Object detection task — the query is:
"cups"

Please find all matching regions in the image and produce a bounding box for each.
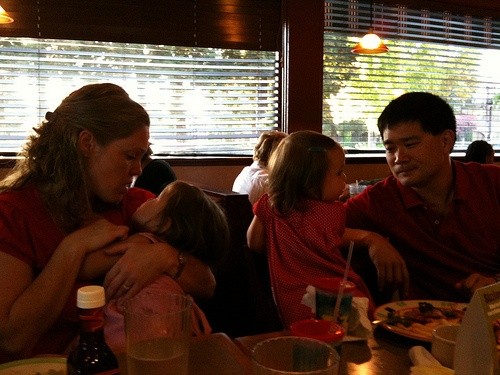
[251,337,340,375]
[431,323,461,369]
[122,294,190,375]
[310,278,356,335]
[349,184,366,198]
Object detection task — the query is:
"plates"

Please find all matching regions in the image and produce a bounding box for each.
[374,300,469,344]
[0,357,67,375]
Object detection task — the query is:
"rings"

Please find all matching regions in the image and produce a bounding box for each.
[121,283,132,290]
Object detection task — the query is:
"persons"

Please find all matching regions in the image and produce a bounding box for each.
[245,130,377,332]
[231,131,289,197]
[131,145,178,196]
[463,139,495,164]
[340,90,500,304]
[61,179,229,355]
[0,81,217,363]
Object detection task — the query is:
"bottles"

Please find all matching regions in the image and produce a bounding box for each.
[67,285,122,375]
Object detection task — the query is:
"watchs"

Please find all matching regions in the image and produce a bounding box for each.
[173,251,189,281]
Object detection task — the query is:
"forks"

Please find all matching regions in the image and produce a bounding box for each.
[372,311,432,326]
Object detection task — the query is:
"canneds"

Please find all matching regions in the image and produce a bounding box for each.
[291,318,346,372]
[314,277,355,325]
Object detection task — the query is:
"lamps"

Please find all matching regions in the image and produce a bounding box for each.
[0,4,14,24]
[349,0,388,54]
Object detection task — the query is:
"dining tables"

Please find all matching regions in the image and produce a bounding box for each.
[112,320,414,375]
